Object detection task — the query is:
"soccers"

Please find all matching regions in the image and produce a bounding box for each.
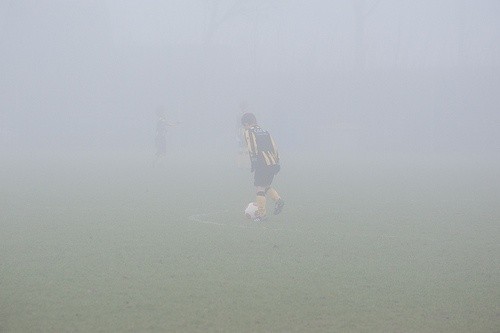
[245,201,264,220]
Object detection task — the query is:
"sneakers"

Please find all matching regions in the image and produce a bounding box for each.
[273,199,285,215]
[252,209,268,223]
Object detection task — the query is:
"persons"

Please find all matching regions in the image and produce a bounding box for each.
[241,113,285,223]
